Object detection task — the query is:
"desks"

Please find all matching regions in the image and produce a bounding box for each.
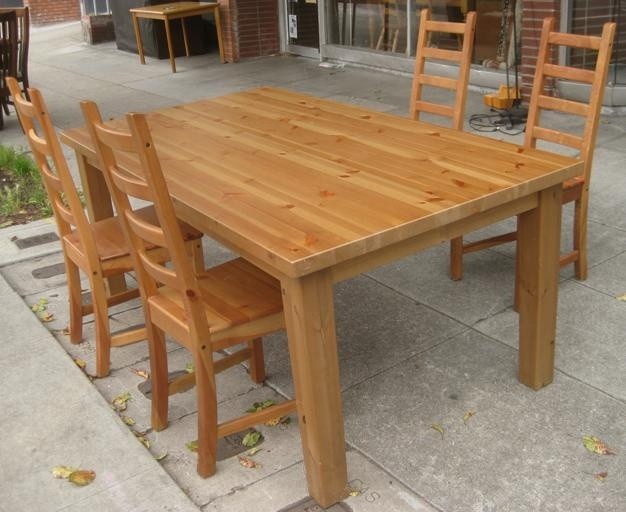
[128,2,226,74]
[57,86,586,509]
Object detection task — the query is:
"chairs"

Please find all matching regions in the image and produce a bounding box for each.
[375,0,433,52]
[0,6,32,130]
[80,99,297,478]
[407,8,476,132]
[449,15,615,312]
[7,77,206,379]
